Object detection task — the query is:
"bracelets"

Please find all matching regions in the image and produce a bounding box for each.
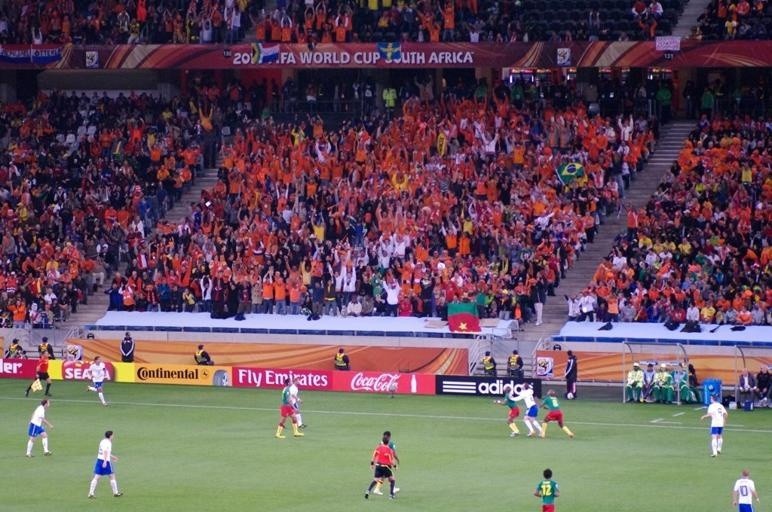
[756,498,759,501]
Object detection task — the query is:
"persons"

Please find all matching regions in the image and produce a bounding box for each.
[289,378,307,429]
[508,382,542,438]
[86,430,124,500]
[0,1,772,407]
[533,469,562,512]
[492,387,521,437]
[25,398,53,458]
[364,435,398,500]
[539,388,574,439]
[372,432,401,496]
[732,468,761,512]
[274,378,305,439]
[700,395,728,456]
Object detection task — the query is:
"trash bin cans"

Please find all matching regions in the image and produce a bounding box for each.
[704,379,723,405]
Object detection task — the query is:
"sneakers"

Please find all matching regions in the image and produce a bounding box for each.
[21,384,111,409]
[506,430,576,439]
[626,394,684,406]
[86,491,125,499]
[272,422,309,440]
[363,486,402,501]
[23,450,56,459]
[708,447,724,458]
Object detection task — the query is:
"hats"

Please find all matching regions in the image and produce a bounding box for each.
[631,361,685,369]
[12,337,48,344]
[125,332,130,336]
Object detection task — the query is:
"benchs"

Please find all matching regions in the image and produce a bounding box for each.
[541,379,737,390]
